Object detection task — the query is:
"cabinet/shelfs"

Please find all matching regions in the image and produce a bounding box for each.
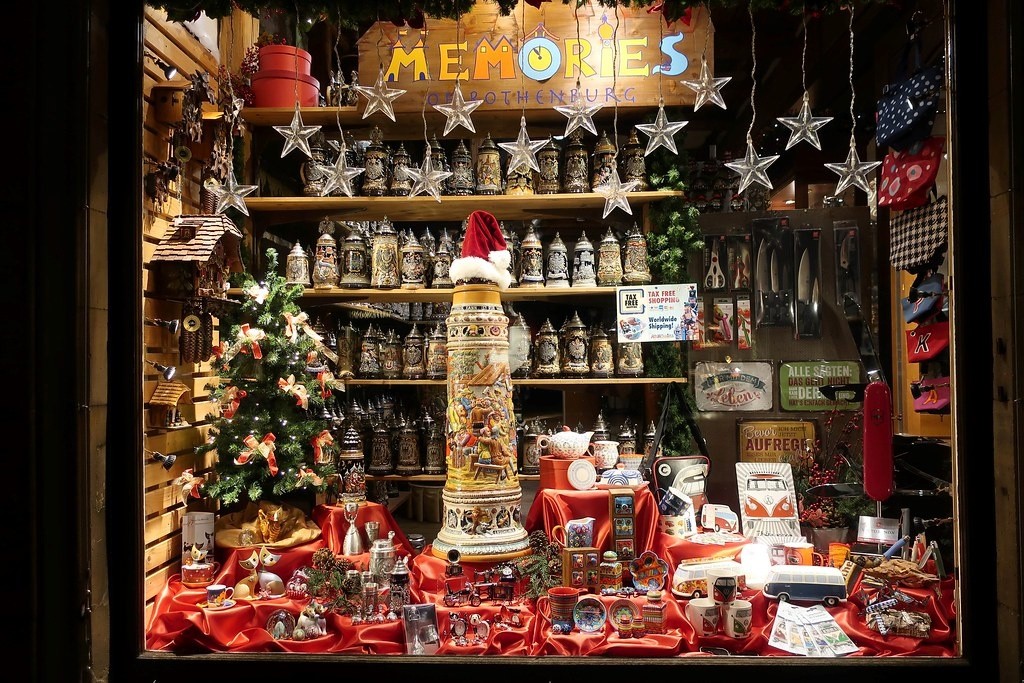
[144,1,969,670]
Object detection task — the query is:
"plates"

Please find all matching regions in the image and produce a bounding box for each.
[181,578,214,588]
[199,599,237,611]
[566,459,597,490]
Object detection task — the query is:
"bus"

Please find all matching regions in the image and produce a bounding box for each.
[672,562,747,599]
[763,566,848,608]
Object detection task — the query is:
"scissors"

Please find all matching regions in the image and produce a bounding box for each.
[705,238,725,288]
[728,241,750,289]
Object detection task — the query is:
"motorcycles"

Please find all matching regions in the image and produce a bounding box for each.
[443,582,481,607]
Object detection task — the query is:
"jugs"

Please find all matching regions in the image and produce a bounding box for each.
[537,426,595,460]
[587,441,620,468]
[551,517,595,547]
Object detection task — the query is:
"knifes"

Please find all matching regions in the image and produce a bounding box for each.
[756,238,819,336]
[838,239,849,298]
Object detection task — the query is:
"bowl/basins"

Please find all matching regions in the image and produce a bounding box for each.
[573,597,607,633]
[608,599,642,630]
[630,551,669,591]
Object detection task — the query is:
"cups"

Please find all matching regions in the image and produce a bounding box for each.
[784,542,824,566]
[658,515,685,540]
[206,585,234,608]
[619,454,644,470]
[706,568,737,606]
[537,588,580,634]
[364,522,380,544]
[723,600,753,639]
[181,562,220,583]
[684,598,719,637]
[654,487,693,516]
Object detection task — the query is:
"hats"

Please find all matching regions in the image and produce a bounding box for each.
[449,209,512,291]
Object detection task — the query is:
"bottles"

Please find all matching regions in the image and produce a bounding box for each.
[619,616,632,638]
[642,591,668,634]
[632,616,645,638]
[599,551,623,595]
